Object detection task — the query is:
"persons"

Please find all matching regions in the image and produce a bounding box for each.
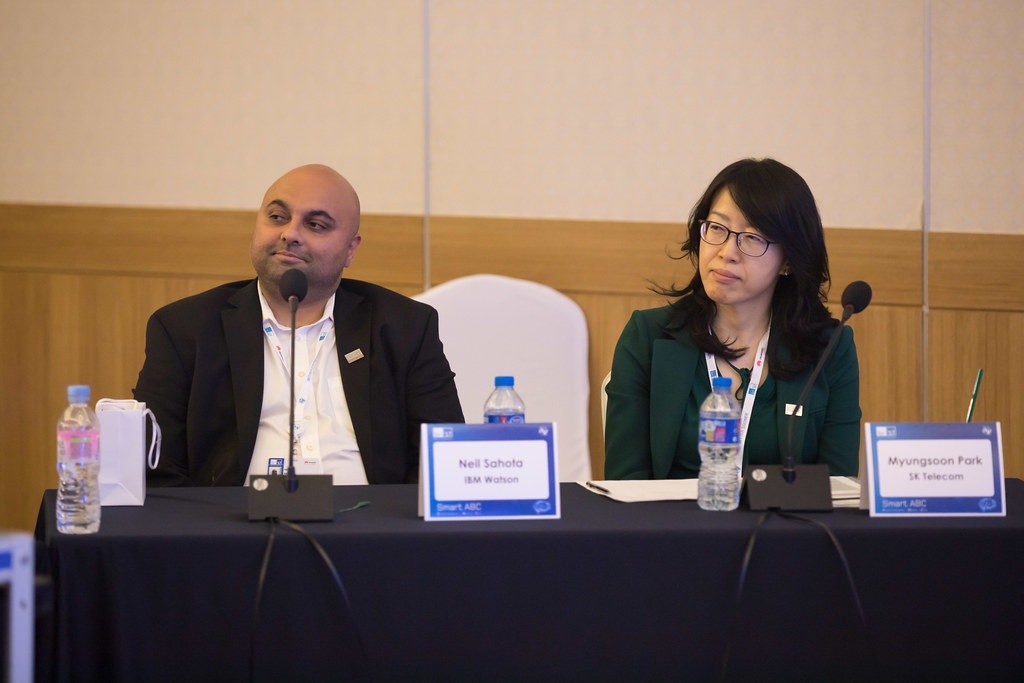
[603,158,863,482]
[130,163,465,487]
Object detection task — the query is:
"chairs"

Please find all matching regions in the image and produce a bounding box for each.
[407,271,591,483]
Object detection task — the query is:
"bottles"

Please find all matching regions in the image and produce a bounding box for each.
[696,378,746,511]
[54,385,103,534]
[483,377,526,426]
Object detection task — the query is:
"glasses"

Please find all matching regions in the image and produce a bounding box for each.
[698,219,783,257]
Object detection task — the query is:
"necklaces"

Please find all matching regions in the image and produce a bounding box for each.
[710,324,756,407]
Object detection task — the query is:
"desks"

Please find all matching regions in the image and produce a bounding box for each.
[33,475,1023,683]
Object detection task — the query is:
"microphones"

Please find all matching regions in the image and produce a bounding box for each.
[246,269,336,525]
[739,279,874,510]
